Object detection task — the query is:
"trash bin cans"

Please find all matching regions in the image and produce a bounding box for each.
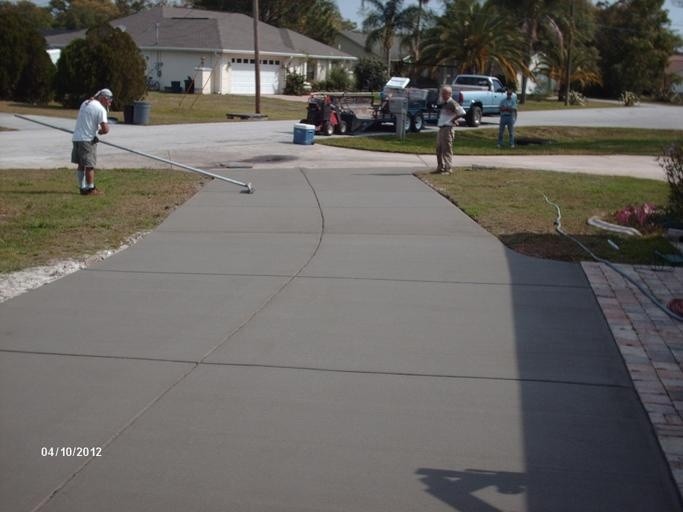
[124,100,150,124]
[172,81,180,93]
[184,80,194,93]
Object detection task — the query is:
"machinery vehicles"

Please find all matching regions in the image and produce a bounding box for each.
[298,85,428,136]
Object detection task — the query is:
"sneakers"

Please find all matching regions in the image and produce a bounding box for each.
[80,186,107,196]
[495,143,516,149]
[429,168,455,176]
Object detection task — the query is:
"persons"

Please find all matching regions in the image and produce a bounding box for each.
[429,87,466,175]
[495,86,519,149]
[71,87,115,196]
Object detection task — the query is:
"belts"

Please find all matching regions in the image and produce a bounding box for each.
[439,125,453,128]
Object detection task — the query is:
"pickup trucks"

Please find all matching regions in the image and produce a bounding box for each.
[424,75,517,127]
